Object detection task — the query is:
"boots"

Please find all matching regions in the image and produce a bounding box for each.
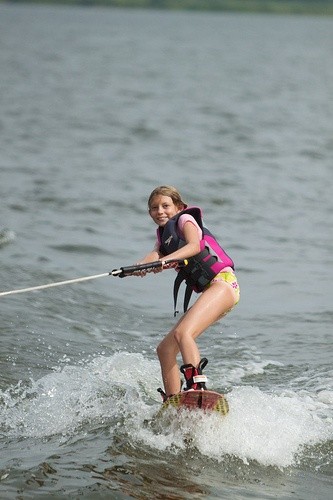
[179,358,208,392]
[157,380,183,402]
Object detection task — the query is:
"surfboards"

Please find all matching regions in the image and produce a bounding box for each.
[148,388,232,420]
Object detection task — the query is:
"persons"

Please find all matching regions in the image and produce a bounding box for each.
[125,184,242,403]
